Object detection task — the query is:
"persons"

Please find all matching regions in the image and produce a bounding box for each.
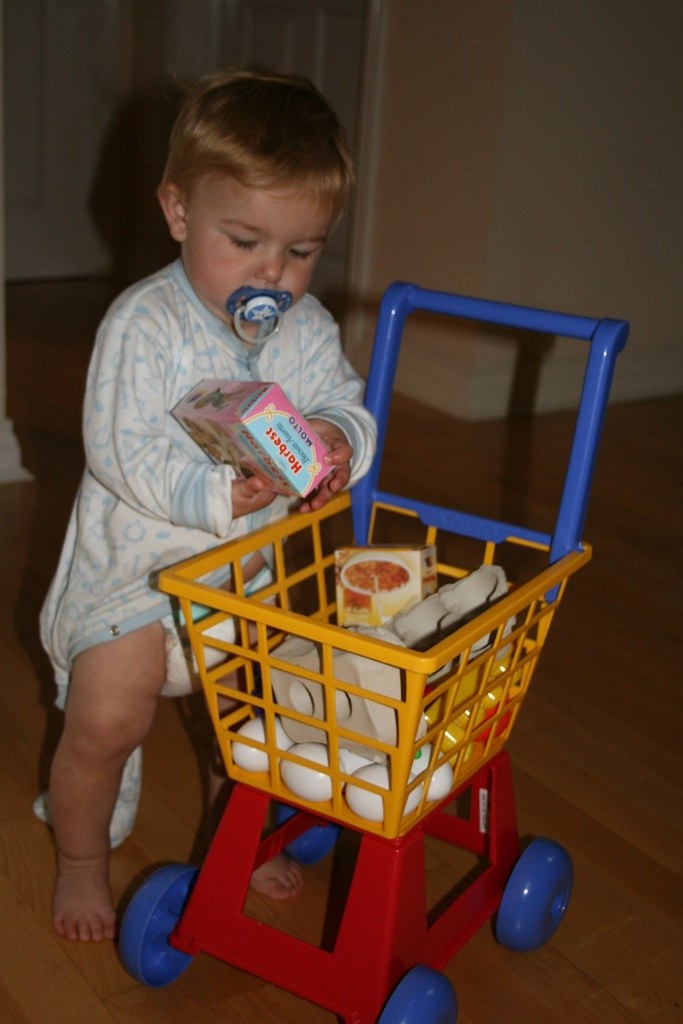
[35,64,376,943]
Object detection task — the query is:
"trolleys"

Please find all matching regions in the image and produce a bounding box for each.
[117,270,631,1023]
